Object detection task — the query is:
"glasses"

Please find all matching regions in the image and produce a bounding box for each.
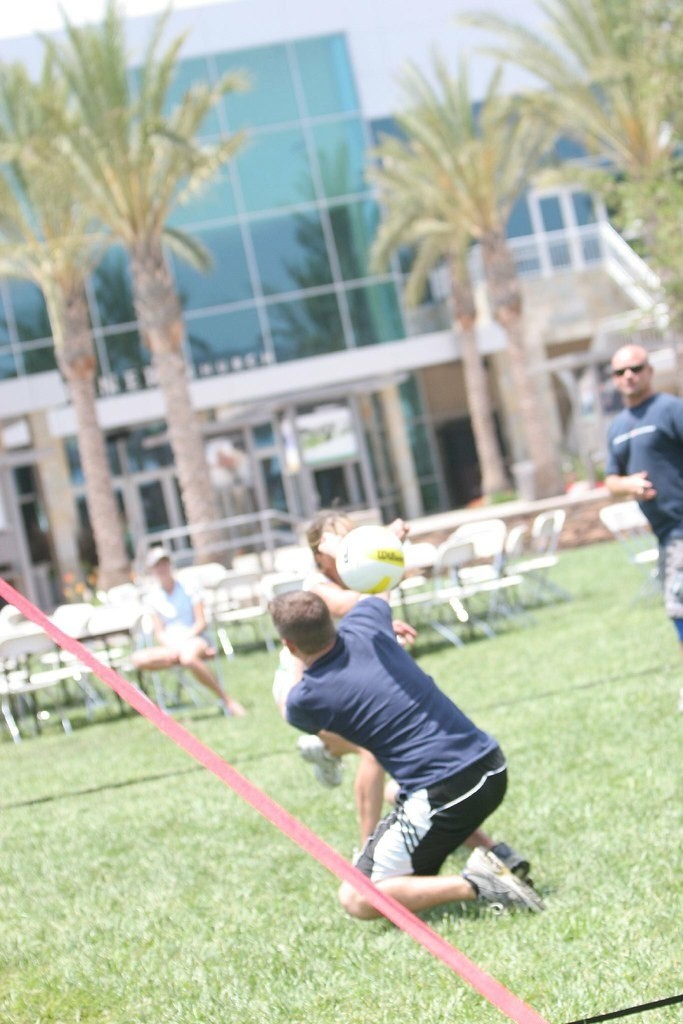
[613,364,646,376]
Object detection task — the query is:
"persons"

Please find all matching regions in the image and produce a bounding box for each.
[267,510,547,918]
[131,547,246,716]
[606,345,683,715]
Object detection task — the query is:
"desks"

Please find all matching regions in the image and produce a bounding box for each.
[25,628,150,737]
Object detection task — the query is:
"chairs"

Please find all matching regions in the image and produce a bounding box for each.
[0,508,567,742]
[599,499,663,591]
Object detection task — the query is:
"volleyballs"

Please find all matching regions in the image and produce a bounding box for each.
[334,523,408,596]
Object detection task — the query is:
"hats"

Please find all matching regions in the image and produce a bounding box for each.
[147,547,172,568]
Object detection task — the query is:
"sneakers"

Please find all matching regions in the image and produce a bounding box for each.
[489,841,534,888]
[460,844,547,916]
[296,735,344,790]
